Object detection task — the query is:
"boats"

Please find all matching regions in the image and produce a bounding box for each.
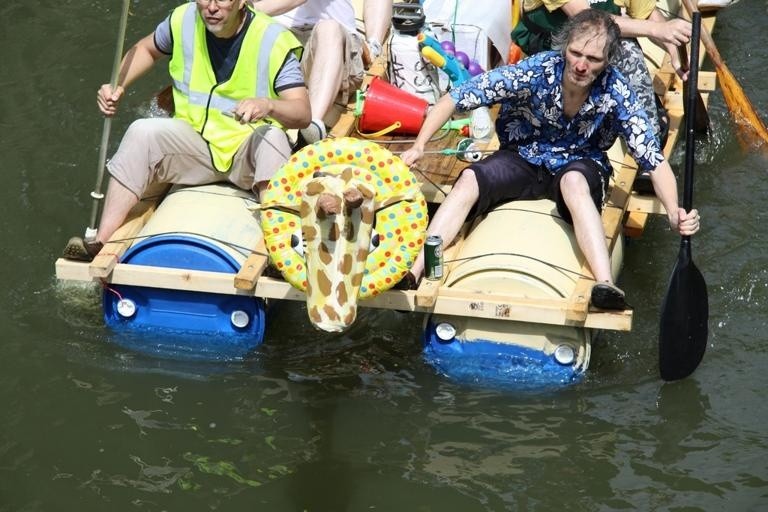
[55,3,721,397]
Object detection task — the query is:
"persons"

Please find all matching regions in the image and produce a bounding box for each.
[60,1,314,261]
[248,0,738,200]
[389,7,703,312]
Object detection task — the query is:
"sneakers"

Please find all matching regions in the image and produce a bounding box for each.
[590,282,626,310]
[296,119,326,147]
[62,237,103,262]
[391,272,417,290]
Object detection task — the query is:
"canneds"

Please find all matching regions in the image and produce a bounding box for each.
[455,138,482,163]
[424,235,444,281]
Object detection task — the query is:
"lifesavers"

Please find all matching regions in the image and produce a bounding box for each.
[260,137,429,334]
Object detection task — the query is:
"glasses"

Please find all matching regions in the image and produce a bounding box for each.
[196,0,233,6]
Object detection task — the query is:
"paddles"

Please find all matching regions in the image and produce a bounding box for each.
[676,36,713,136]
[682,0,768,156]
[660,13,708,380]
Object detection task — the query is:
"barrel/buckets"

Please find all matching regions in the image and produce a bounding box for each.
[423,273,591,393]
[103,239,267,361]
[353,74,428,140]
